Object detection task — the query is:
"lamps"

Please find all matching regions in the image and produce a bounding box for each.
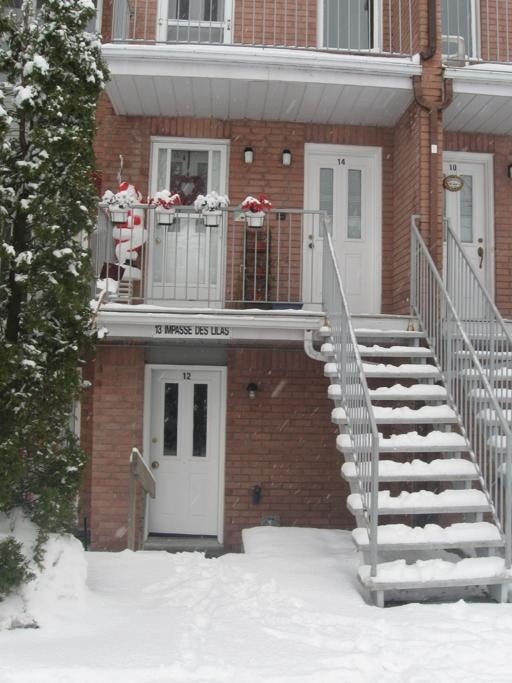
[246,383,257,399]
[282,150,291,166]
[244,147,253,163]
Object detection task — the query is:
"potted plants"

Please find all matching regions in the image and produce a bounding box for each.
[194,191,230,227]
[147,189,182,225]
[98,189,133,224]
[240,193,272,228]
